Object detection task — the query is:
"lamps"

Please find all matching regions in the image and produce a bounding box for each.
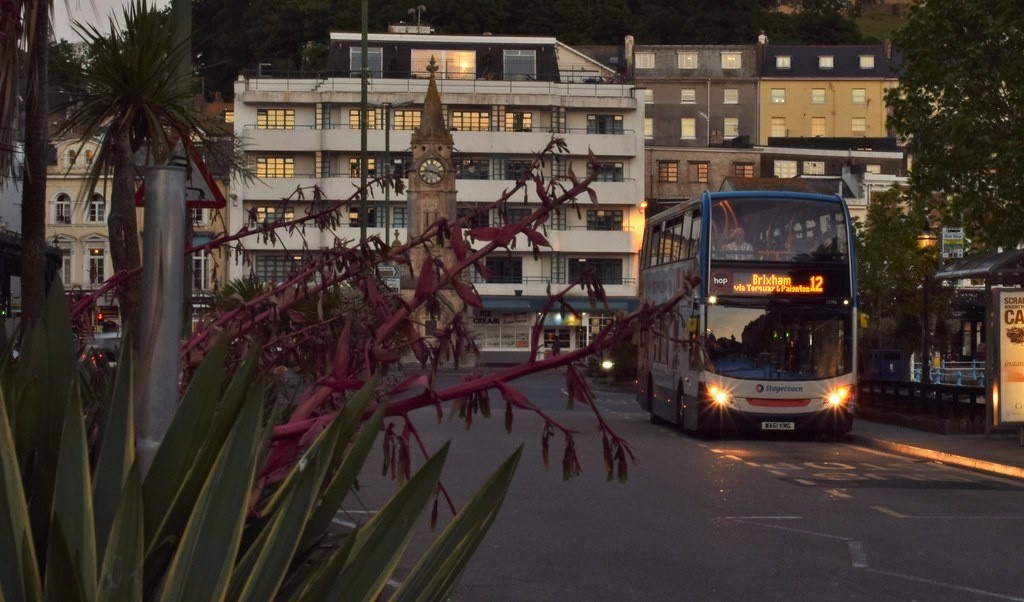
[230,192,239,207]
[640,200,647,214]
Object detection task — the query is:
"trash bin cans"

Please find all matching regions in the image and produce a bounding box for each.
[863,348,906,380]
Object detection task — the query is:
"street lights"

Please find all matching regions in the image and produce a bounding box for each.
[915,229,938,385]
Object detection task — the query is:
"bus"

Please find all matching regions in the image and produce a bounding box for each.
[633,189,860,438]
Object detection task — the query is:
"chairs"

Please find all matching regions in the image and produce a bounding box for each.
[789,239,815,260]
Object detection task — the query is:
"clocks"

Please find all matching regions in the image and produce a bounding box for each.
[418,158,445,184]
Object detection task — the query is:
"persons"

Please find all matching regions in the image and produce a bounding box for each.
[550,333,561,357]
[722,228,755,260]
